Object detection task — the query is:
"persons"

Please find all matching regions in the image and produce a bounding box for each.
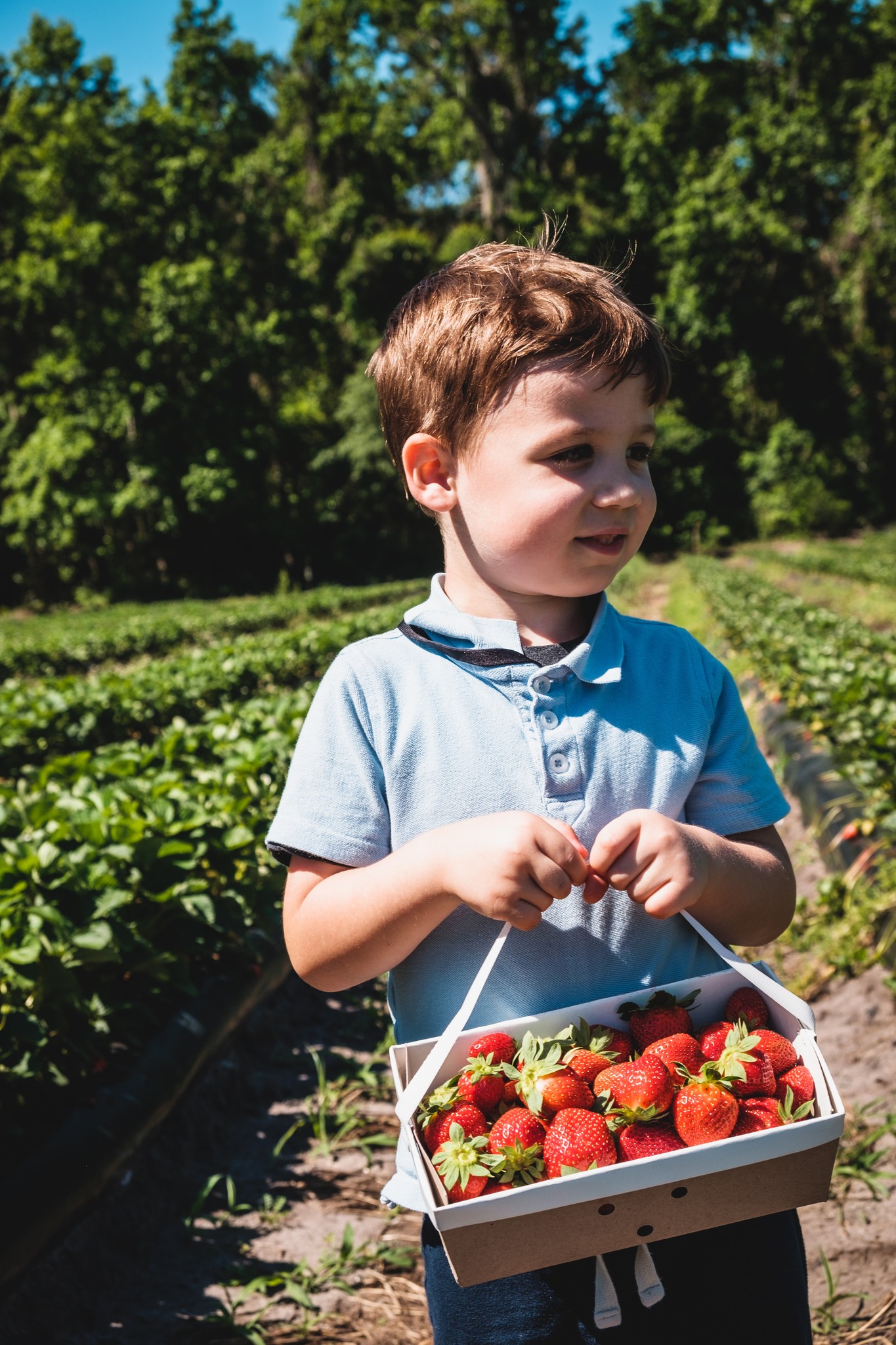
[264,240,813,1345]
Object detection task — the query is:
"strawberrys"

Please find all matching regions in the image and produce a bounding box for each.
[416,987,817,1201]
[756,678,875,840]
[90,951,262,1073]
